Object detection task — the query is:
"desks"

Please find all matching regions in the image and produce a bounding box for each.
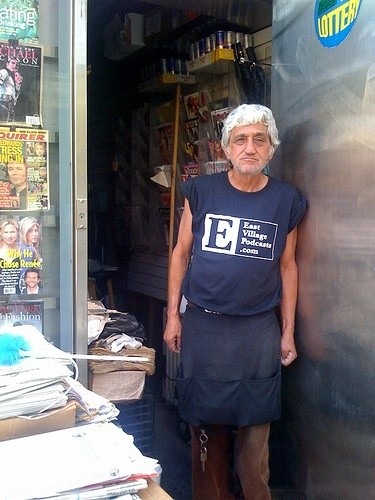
[127,249,169,347]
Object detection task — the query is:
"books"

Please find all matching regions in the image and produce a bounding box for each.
[150,91,229,247]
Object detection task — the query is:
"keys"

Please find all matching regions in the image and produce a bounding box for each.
[200,434,208,472]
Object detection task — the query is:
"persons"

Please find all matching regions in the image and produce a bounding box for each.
[163,104,307,500]
[0,57,48,294]
[193,152,199,162]
[186,143,195,165]
[215,141,223,161]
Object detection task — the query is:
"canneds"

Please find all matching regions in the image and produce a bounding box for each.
[159,58,167,74]
[167,58,176,75]
[175,59,183,74]
[189,30,252,60]
